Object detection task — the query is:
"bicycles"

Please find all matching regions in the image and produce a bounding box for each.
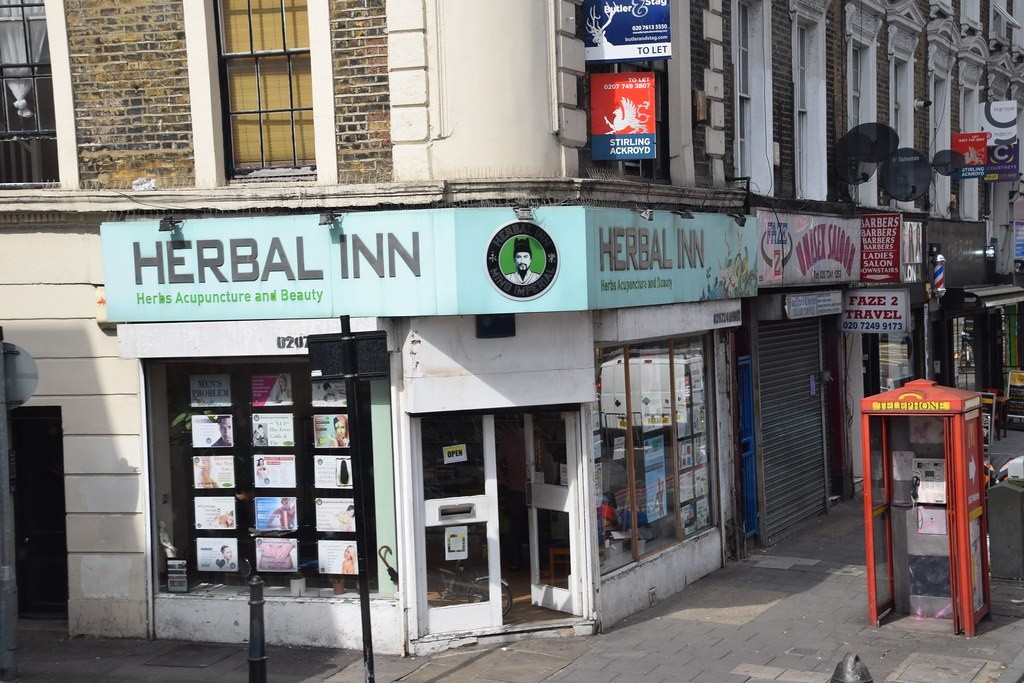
[426,560,512,617]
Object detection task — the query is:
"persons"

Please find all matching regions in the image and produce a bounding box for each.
[158,353,376,584]
[505,237,541,285]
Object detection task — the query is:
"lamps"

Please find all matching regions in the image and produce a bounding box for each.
[932,253,947,297]
[985,245,995,257]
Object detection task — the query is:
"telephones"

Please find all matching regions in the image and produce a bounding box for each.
[910,457,948,505]
